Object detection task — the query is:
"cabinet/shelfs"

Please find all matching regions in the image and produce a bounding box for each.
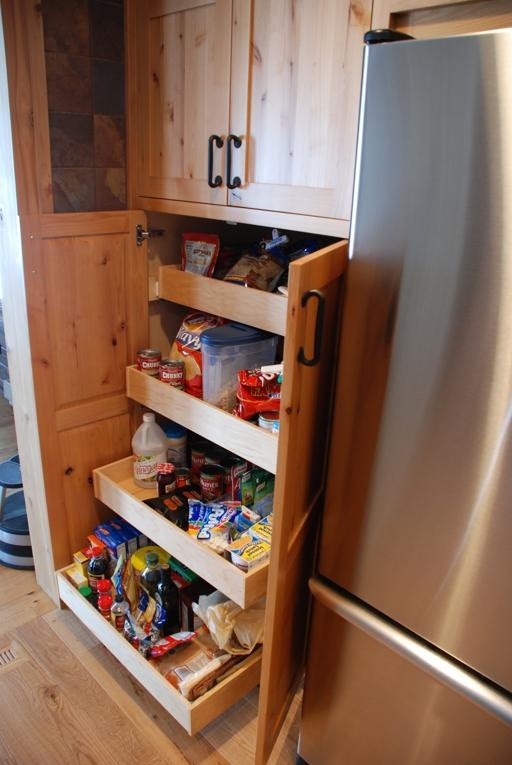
[25,198,348,765]
[126,2,373,240]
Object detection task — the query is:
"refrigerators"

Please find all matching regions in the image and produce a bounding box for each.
[292,25,511,764]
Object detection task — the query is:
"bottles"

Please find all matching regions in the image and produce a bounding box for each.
[110,594,129,632]
[151,564,180,638]
[140,553,161,596]
[87,548,109,610]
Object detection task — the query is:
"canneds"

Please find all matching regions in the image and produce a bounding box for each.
[159,359,186,392]
[136,350,162,379]
[172,442,242,505]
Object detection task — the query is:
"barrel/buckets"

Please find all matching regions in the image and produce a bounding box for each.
[130,413,168,489]
